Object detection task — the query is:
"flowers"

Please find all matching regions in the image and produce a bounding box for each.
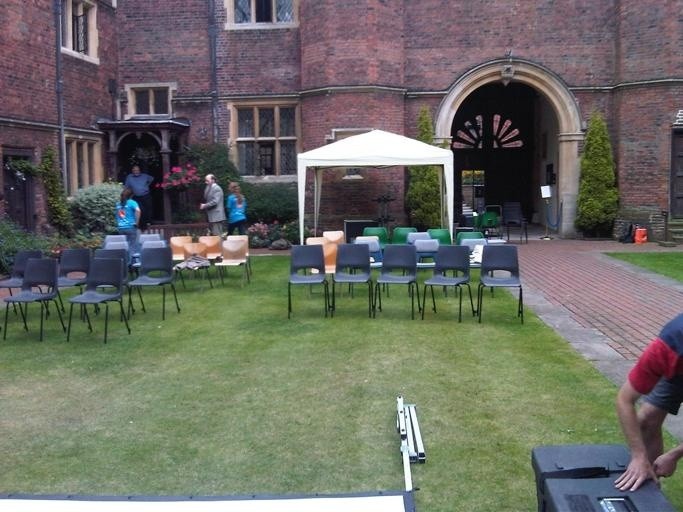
[156,161,202,192]
[247,218,271,238]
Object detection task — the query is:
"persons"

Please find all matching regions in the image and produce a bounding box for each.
[226,181,248,234]
[612,314,683,494]
[124,164,153,230]
[198,173,226,237]
[115,188,140,266]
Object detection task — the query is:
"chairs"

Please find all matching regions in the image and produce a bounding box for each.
[283,202,528,324]
[1,231,252,346]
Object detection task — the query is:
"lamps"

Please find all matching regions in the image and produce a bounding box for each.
[539,185,554,241]
